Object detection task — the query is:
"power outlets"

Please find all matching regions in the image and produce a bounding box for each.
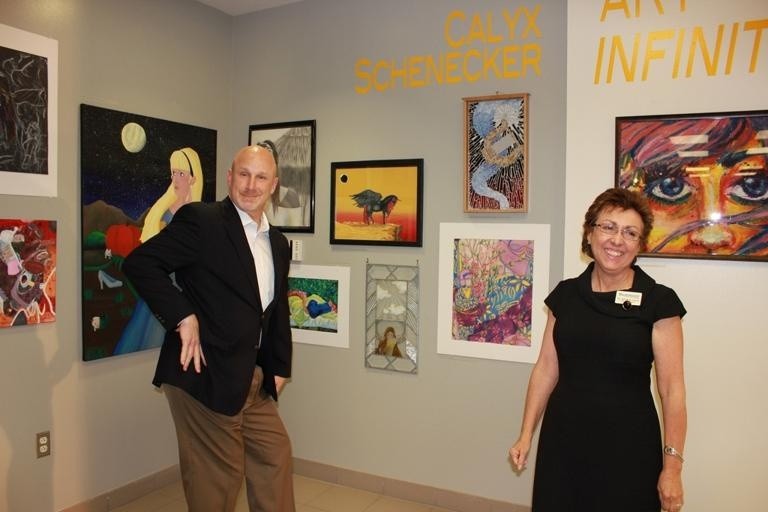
[37,432,50,458]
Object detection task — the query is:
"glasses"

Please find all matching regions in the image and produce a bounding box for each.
[589,219,647,243]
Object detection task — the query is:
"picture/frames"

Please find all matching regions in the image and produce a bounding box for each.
[0,23,60,198]
[330,158,425,247]
[287,264,351,349]
[248,119,316,232]
[365,264,420,375]
[463,93,529,214]
[436,222,552,363]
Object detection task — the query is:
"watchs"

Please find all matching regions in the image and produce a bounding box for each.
[664,445,685,463]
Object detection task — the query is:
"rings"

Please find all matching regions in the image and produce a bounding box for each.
[677,503,682,507]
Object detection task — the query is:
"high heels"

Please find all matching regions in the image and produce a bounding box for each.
[96,269,124,291]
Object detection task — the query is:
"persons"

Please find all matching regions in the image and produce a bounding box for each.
[509,188,687,512]
[122,145,296,512]
[113,147,204,356]
[376,327,403,358]
